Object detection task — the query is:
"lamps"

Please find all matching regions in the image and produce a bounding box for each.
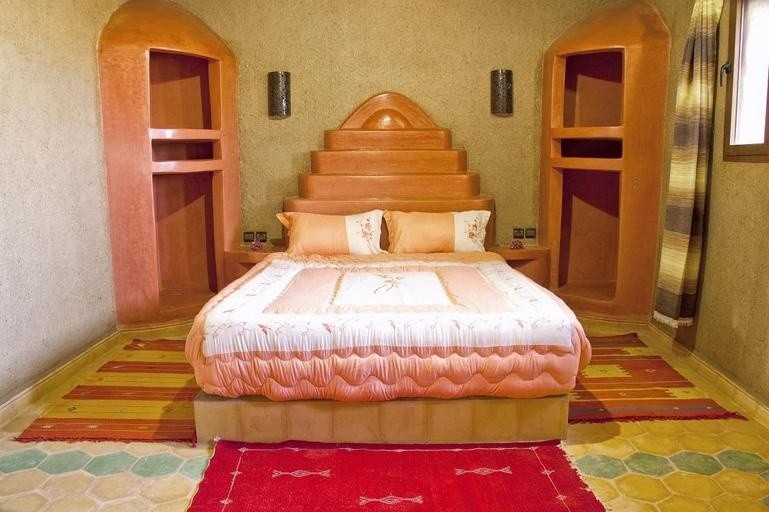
[491,69,512,117]
[267,71,291,119]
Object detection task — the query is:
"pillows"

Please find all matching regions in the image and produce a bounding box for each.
[383,209,492,253]
[275,209,388,256]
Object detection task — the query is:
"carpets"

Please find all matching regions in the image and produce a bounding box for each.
[185,435,609,512]
[12,337,202,443]
[569,332,748,422]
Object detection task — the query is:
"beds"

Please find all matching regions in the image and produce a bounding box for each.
[184,90,593,446]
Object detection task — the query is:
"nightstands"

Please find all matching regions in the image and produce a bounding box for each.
[495,245,550,291]
[224,246,285,287]
